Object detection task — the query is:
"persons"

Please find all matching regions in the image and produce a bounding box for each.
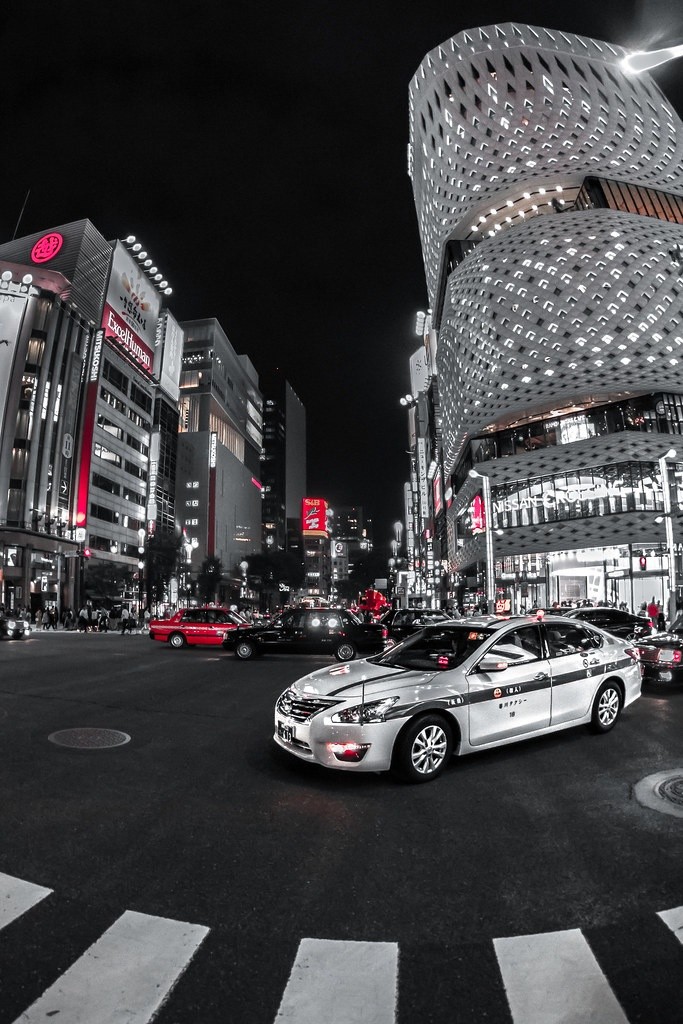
[210,603,495,627]
[519,596,668,634]
[0,602,179,635]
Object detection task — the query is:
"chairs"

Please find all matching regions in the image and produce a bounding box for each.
[455,629,585,665]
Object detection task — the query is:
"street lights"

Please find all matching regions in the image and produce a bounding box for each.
[467,470,495,616]
[659,449,679,626]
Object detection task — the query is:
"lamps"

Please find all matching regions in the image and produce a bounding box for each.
[0,269,33,295]
[398,393,418,407]
[413,310,427,336]
[120,234,175,297]
[425,460,438,481]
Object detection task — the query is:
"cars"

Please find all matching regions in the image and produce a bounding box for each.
[0,613,31,640]
[275,612,641,782]
[546,607,652,646]
[222,605,389,663]
[150,607,253,650]
[634,615,682,684]
[376,608,459,654]
[525,606,578,617]
